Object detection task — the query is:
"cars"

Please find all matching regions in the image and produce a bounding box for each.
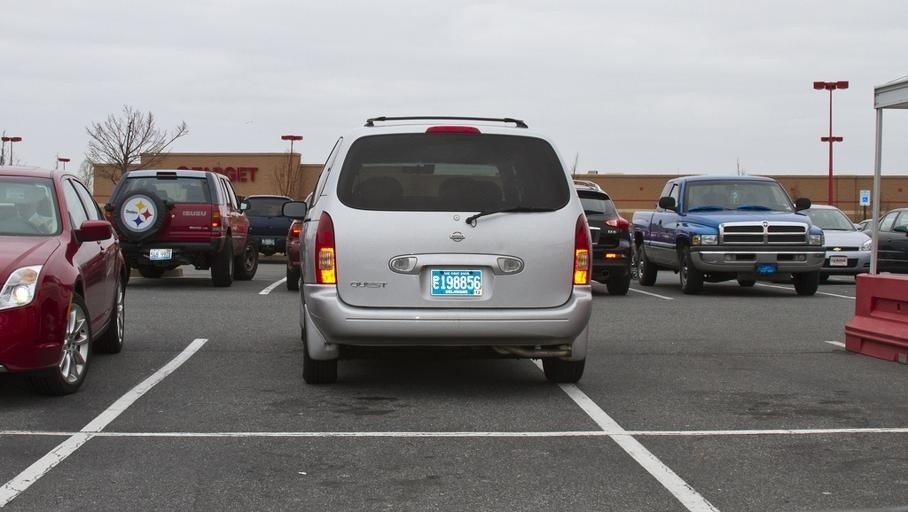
[793,204,871,281]
[857,219,872,239]
[241,194,293,256]
[285,192,314,291]
[0,166,126,395]
[861,208,907,273]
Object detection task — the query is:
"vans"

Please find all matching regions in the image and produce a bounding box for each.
[282,115,596,386]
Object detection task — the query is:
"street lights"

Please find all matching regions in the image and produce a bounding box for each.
[280,135,303,154]
[57,158,71,170]
[1,137,21,166]
[812,81,848,204]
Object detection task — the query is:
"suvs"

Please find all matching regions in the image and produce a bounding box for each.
[103,169,259,288]
[572,179,630,296]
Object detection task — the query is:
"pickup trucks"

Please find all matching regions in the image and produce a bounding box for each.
[630,173,824,296]
